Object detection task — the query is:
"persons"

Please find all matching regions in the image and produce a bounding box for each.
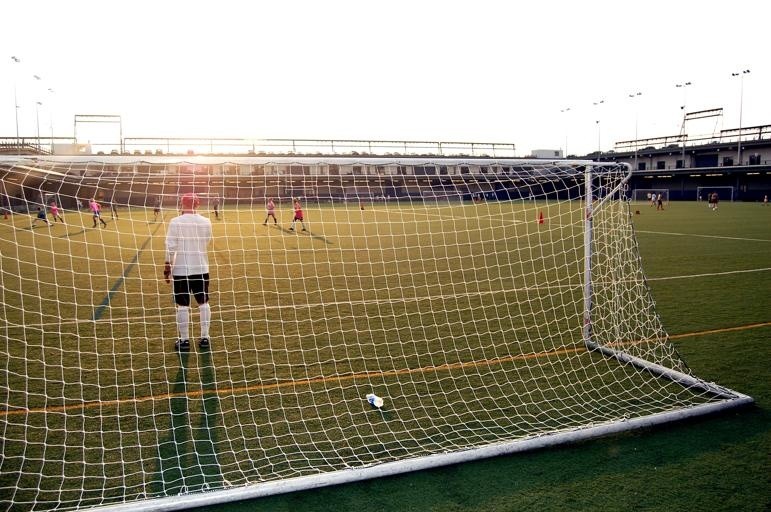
[712,190,720,211]
[152,197,162,223]
[212,193,220,220]
[32,207,54,227]
[48,197,65,223]
[163,191,215,349]
[656,192,665,210]
[648,192,653,205]
[699,190,703,202]
[651,191,658,208]
[289,196,308,231]
[109,196,120,220]
[86,198,108,229]
[528,190,533,201]
[263,197,278,226]
[708,191,714,208]
[470,192,489,203]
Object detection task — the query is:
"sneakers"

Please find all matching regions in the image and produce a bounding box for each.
[175,339,190,350]
[199,338,209,348]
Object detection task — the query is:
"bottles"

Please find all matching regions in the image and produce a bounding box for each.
[364,393,384,408]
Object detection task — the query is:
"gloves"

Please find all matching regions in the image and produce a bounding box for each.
[164,262,171,283]
